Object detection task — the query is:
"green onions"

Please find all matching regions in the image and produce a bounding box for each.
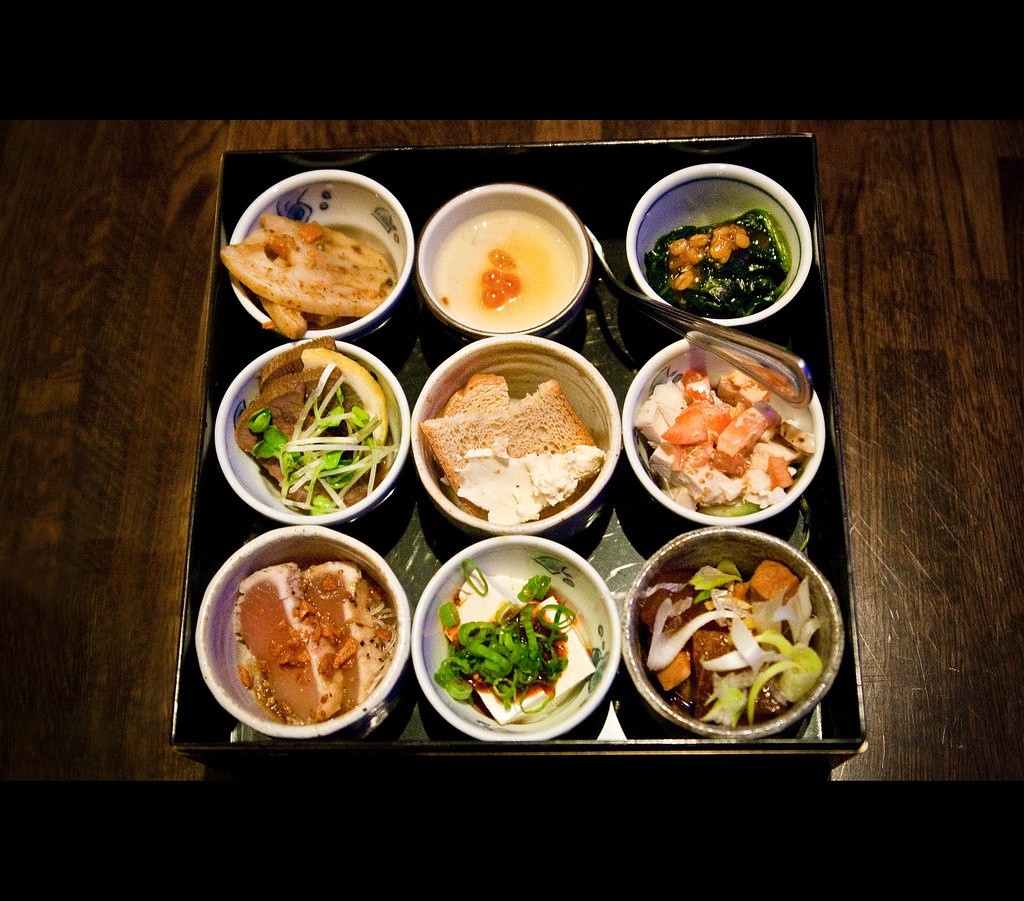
[641,560,822,728]
[435,559,577,714]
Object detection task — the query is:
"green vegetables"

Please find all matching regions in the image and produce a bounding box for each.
[645,209,792,318]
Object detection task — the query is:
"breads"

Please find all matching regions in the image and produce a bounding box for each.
[418,373,604,525]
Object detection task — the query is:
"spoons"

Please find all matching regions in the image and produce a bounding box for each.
[583,223,813,406]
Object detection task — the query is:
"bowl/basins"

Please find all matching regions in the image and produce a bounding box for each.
[412,535,621,741]
[213,337,411,524]
[411,334,622,535]
[228,167,415,338]
[194,524,412,739]
[415,181,593,336]
[622,338,826,526]
[622,526,845,739]
[624,162,813,327]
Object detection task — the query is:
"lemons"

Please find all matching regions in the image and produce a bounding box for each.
[300,347,387,446]
